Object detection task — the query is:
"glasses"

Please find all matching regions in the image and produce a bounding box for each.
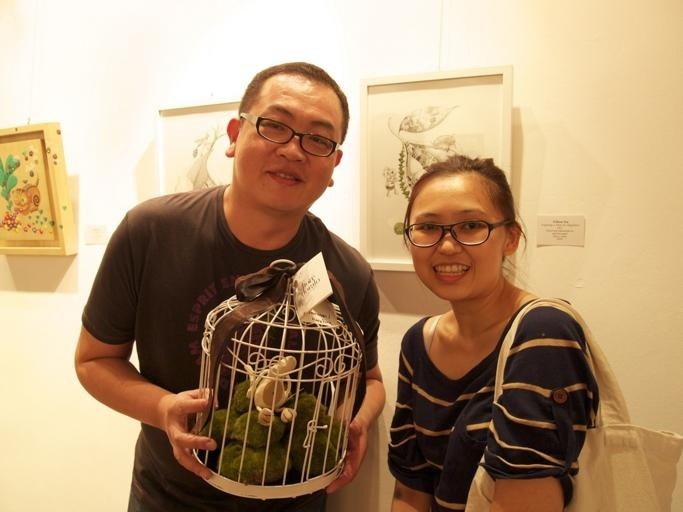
[405,218,515,247]
[240,110,340,158]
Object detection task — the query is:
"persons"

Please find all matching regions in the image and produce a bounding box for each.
[385,151,599,512]
[74,61,386,511]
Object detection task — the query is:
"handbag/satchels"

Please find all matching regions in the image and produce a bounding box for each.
[466,423,683,512]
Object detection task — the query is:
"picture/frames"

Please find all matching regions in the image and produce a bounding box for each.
[354,62,517,275]
[0,120,79,260]
[152,98,248,203]
